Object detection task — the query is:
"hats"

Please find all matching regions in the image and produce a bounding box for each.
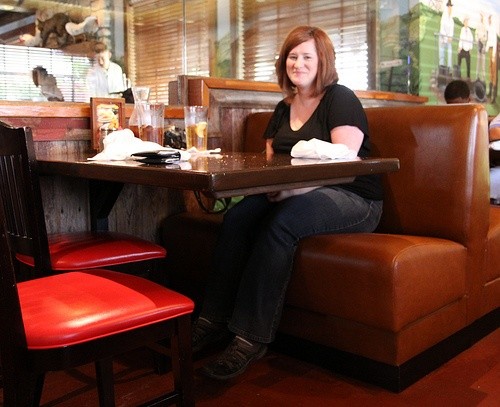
[85,127,163,162]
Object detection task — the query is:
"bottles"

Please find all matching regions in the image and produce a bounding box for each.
[129,87,152,141]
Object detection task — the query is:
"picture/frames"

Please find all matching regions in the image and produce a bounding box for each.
[90,97,125,156]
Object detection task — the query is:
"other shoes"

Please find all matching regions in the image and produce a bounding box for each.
[204,335,269,382]
[191,312,224,354]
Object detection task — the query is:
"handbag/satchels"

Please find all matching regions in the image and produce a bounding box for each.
[163,126,186,150]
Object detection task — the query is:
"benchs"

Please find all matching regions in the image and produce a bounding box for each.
[160,102,500,393]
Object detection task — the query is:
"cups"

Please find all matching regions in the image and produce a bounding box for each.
[184,105,208,150]
[98,128,117,151]
[137,102,164,145]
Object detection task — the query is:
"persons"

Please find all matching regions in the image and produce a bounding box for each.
[33,66,65,101]
[191,26,383,380]
[445,80,500,204]
[85,49,124,105]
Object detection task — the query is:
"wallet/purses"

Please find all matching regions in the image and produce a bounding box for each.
[132,147,181,165]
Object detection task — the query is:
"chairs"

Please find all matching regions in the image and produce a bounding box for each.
[0,122,194,407]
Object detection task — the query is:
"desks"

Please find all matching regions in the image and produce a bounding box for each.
[35,150,399,233]
